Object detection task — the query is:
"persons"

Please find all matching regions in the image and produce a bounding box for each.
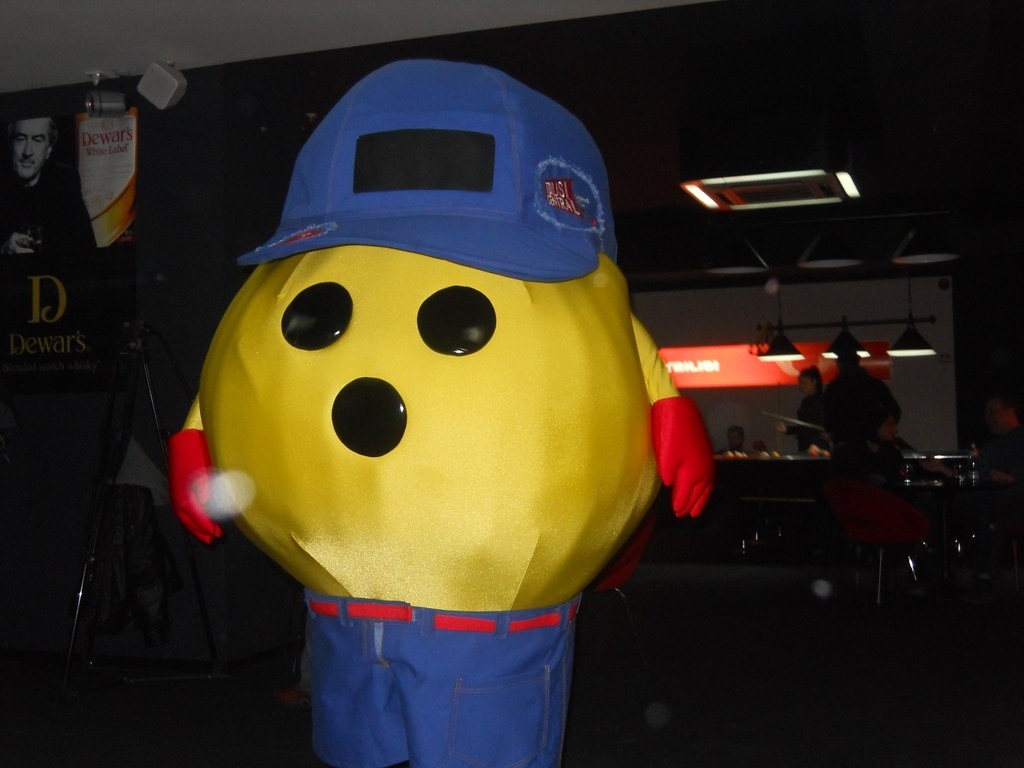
[166,57,717,768]
[811,349,901,455]
[818,411,930,595]
[919,391,1024,595]
[0,116,84,303]
[775,366,831,451]
[716,425,747,454]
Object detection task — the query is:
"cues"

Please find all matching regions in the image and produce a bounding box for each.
[760,409,824,433]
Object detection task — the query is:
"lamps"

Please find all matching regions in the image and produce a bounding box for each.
[750,286,809,363]
[886,279,937,359]
[820,281,871,361]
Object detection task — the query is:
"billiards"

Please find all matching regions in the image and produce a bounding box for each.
[723,444,830,458]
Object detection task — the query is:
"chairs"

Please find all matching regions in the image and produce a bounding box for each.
[821,478,944,604]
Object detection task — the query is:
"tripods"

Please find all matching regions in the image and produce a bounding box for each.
[58,317,222,692]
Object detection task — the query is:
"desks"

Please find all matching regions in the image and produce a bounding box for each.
[899,473,1007,593]
